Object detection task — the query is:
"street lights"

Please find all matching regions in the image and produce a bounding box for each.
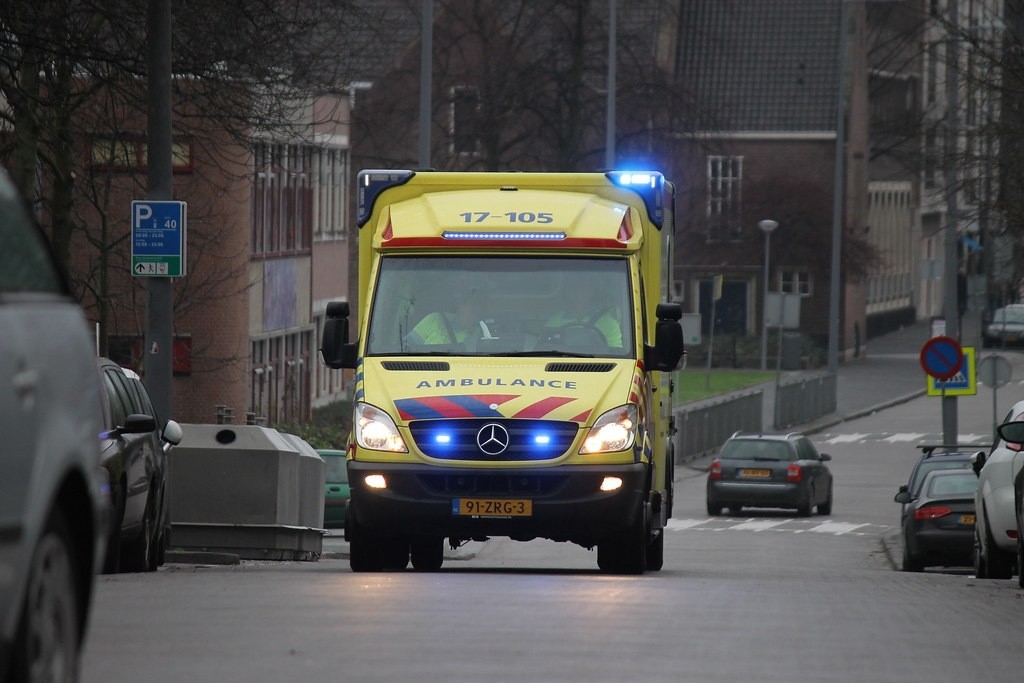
[756,217,778,371]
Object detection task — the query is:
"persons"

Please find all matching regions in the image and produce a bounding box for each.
[545,279,624,348]
[401,287,486,345]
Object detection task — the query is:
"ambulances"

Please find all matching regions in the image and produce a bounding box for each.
[321,167,686,578]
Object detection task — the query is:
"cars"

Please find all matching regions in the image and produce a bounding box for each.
[895,403,1023,589]
[0,149,104,683]
[95,357,184,575]
[983,304,1024,347]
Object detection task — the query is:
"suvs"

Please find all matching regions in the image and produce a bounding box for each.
[707,432,835,518]
